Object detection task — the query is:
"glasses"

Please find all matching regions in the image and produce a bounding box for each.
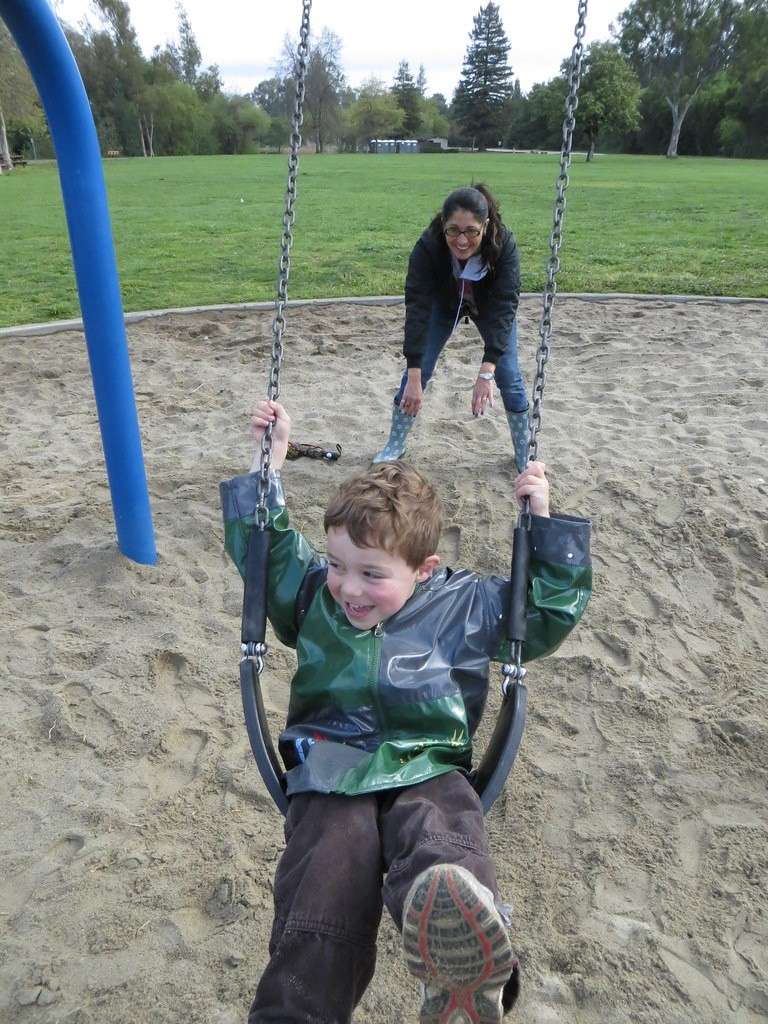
[444,221,484,239]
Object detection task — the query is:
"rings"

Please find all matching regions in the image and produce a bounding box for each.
[481,398,487,400]
[406,403,409,407]
[401,399,405,402]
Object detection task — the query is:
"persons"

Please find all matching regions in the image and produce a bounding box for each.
[371,176,530,474]
[221,394,592,1024]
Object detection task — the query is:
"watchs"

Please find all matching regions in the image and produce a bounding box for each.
[478,372,494,380]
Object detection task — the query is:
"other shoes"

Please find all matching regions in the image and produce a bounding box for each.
[402,864,515,1024]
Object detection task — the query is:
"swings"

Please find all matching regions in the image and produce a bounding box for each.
[241,0,589,818]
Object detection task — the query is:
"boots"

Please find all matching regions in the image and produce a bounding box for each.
[505,408,530,473]
[372,395,417,464]
